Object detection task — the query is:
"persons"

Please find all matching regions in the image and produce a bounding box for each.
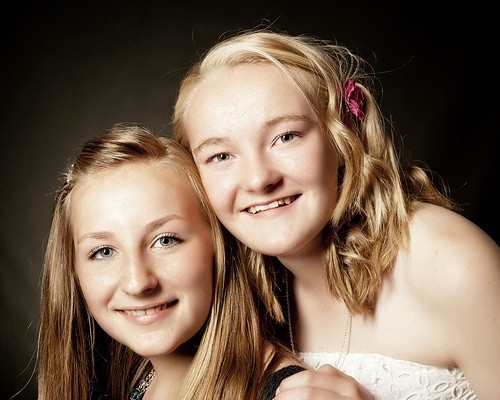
[170,30,500,400]
[38,124,323,400]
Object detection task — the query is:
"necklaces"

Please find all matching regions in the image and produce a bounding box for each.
[130,368,155,400]
[285,236,355,370]
